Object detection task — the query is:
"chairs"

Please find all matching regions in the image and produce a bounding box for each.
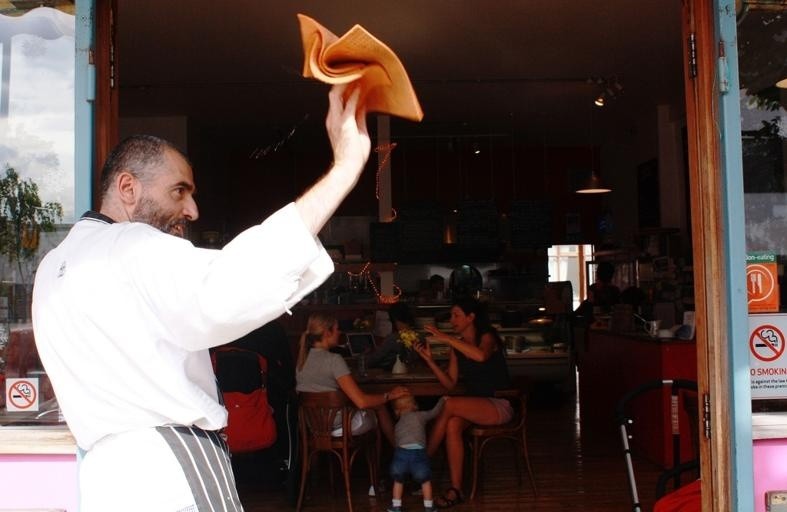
[294,388,384,511]
[618,378,703,512]
[460,377,538,506]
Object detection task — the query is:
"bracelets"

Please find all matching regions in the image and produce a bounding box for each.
[383,390,390,403]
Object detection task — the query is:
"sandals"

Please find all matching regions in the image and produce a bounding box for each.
[434,486,468,509]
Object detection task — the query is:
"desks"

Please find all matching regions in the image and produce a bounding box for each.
[328,367,468,407]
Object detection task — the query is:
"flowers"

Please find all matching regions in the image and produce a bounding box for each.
[378,329,419,356]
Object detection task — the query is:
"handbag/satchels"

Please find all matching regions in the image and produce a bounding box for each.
[215,388,278,454]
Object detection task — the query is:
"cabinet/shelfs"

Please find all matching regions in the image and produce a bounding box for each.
[396,305,576,460]
[281,303,390,337]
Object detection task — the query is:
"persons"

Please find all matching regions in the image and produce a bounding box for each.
[618,286,643,321]
[296,311,410,449]
[411,295,514,508]
[32,82,373,512]
[574,262,618,314]
[428,275,444,298]
[388,395,450,511]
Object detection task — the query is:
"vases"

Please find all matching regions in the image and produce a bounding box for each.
[392,352,412,373]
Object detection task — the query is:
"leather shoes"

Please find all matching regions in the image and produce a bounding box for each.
[386,505,403,511]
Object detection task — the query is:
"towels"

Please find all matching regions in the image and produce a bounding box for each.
[296,14,427,123]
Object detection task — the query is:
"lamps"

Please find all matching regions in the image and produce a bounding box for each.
[579,73,625,108]
[572,109,611,195]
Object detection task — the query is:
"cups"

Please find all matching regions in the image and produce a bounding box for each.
[504,336,530,355]
[643,319,661,338]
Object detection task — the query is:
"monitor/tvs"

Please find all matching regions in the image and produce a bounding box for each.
[346,332,376,356]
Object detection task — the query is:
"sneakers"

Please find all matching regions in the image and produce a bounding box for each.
[368,485,391,497]
